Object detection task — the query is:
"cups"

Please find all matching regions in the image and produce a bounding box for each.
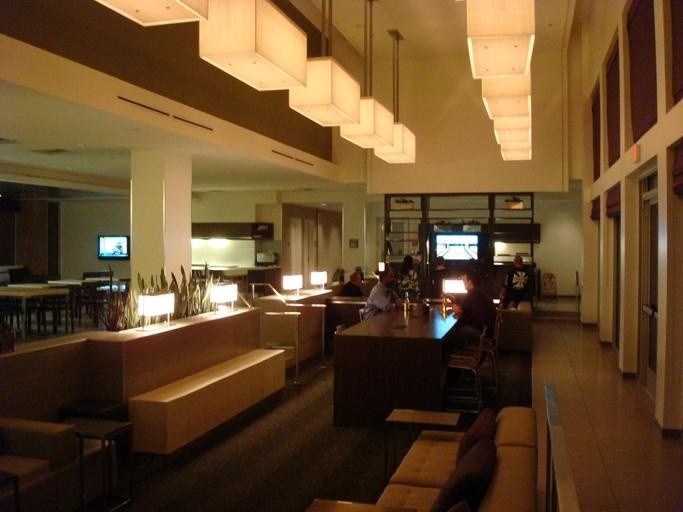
[423,298,430,306]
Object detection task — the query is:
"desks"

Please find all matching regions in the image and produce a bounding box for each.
[0,279,109,340]
[336,308,461,428]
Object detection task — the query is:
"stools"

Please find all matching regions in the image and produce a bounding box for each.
[75,417,133,512]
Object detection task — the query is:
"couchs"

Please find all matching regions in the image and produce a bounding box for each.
[498,301,533,353]
[304,407,538,512]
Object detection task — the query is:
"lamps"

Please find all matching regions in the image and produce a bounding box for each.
[282,274,303,296]
[311,270,327,291]
[465,0,536,164]
[97,0,210,30]
[374,1,415,164]
[288,1,364,127]
[209,282,237,315]
[196,0,309,92]
[136,293,176,332]
[339,1,395,150]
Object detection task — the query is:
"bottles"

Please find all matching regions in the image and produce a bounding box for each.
[403,292,409,311]
[403,311,409,327]
[441,295,447,312]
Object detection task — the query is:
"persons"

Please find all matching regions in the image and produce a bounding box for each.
[336,255,452,320]
[499,255,536,309]
[452,267,497,347]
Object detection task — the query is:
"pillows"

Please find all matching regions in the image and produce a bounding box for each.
[456,410,496,463]
[426,438,497,512]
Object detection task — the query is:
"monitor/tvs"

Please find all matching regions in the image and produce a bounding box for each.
[98,235,129,262]
[435,234,480,261]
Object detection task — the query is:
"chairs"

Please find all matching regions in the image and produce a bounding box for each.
[498,303,504,327]
[359,309,365,323]
[464,315,503,397]
[334,324,345,333]
[445,331,485,413]
[449,323,488,395]
